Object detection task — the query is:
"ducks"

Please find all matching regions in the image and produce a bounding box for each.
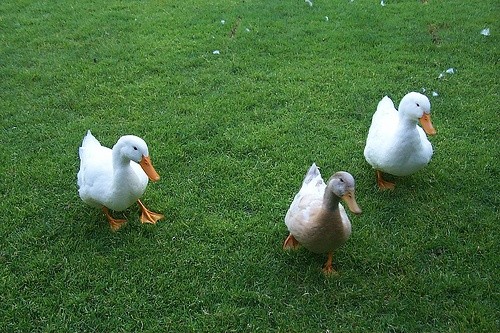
[364,91,437,193]
[76,129,165,234]
[283,162,363,278]
